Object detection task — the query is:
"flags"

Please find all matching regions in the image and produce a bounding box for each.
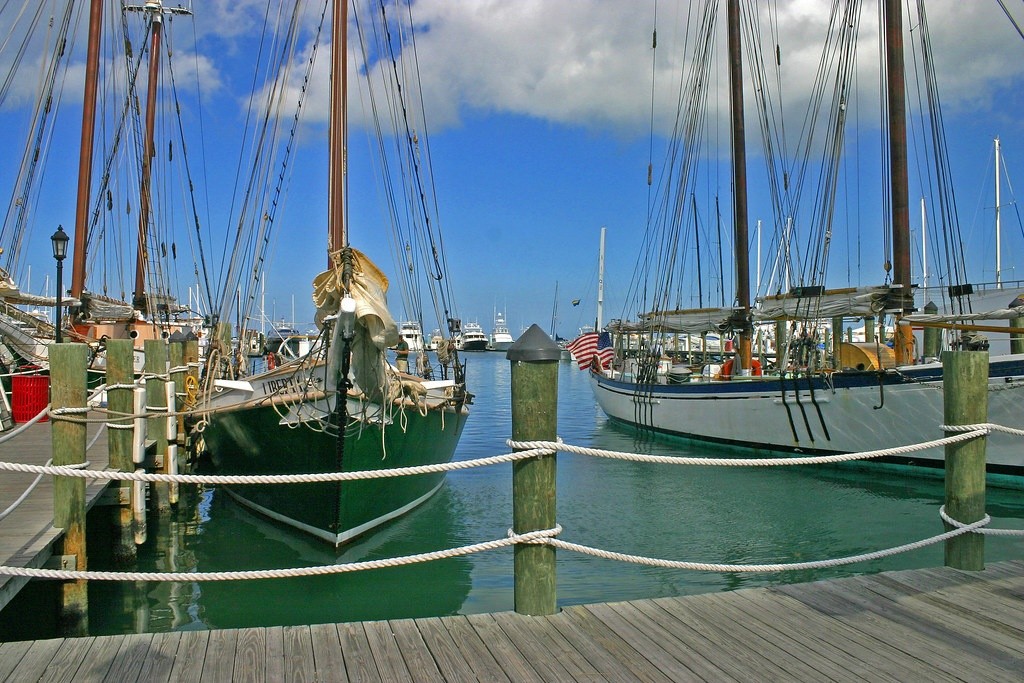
[565,332,614,371]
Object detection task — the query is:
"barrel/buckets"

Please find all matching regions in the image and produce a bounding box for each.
[668,372,693,385]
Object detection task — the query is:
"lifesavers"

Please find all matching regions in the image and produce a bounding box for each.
[267,351,275,370]
[718,358,762,381]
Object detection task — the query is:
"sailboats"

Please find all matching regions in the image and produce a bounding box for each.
[551,1,1024,480]
[1,1,475,550]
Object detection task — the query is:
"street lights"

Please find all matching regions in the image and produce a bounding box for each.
[50,224,69,342]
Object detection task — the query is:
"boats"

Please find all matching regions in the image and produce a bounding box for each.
[399,323,423,351]
[426,330,442,350]
[488,319,514,351]
[454,323,489,352]
[266,321,301,354]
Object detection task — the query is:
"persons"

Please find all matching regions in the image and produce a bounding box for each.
[395,334,408,376]
